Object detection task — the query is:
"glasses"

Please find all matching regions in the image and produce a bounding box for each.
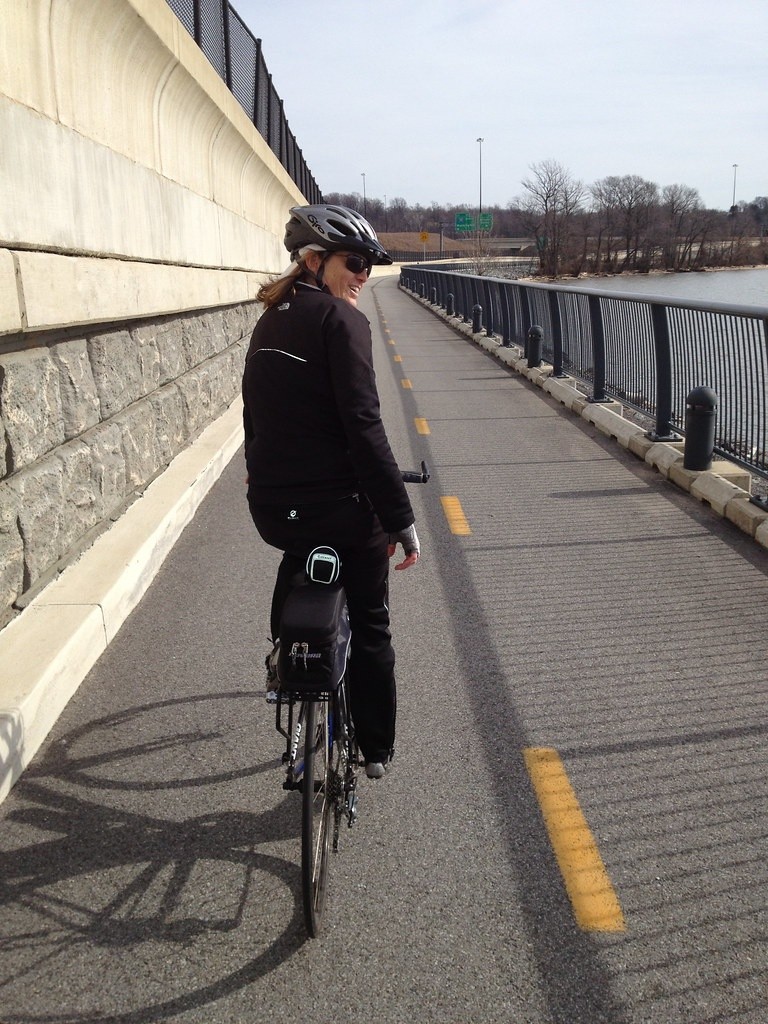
[335,253,372,278]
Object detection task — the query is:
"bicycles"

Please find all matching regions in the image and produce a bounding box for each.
[259,457,431,942]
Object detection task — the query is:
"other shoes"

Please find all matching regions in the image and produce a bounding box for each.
[367,749,392,779]
[263,673,291,705]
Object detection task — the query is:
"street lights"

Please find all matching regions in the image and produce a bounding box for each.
[361,172,368,221]
[729,163,740,235]
[476,137,487,253]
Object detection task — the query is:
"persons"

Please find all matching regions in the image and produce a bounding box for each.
[240,202,419,778]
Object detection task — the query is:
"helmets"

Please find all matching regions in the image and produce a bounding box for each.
[284,203,394,266]
